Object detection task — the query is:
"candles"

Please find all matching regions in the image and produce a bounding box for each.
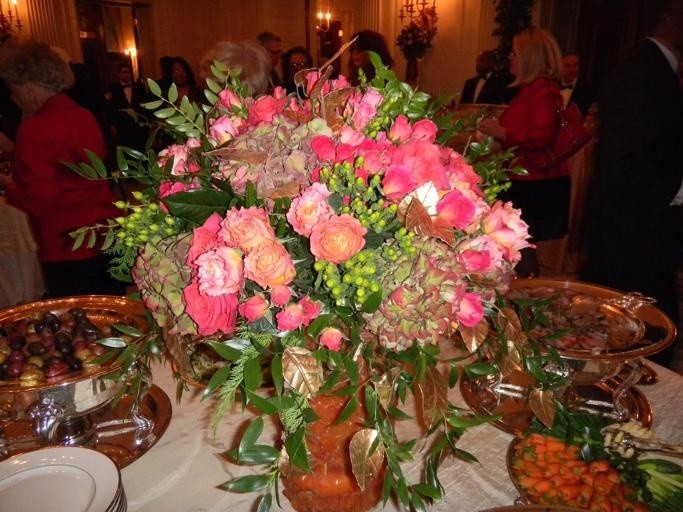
[317,11,324,30]
[14,3,20,21]
[325,11,334,32]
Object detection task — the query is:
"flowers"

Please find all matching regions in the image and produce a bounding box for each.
[63,39,584,510]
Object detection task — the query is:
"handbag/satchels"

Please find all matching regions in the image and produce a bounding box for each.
[521,90,591,170]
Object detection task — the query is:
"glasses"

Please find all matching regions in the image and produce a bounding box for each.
[268,48,282,53]
[290,61,312,70]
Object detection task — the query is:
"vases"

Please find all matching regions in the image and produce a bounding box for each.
[286,333,395,511]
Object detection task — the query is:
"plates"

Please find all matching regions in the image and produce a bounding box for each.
[0,296,173,469]
[0,448,128,511]
[459,277,676,437]
[506,439,527,503]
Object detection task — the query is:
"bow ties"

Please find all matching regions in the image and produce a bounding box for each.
[561,84,573,90]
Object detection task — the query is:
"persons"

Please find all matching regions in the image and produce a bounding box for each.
[282,46,313,96]
[478,27,572,279]
[164,57,199,107]
[256,30,282,90]
[612,1,683,306]
[558,49,599,118]
[107,63,146,148]
[0,41,126,300]
[459,49,504,106]
[347,30,391,90]
